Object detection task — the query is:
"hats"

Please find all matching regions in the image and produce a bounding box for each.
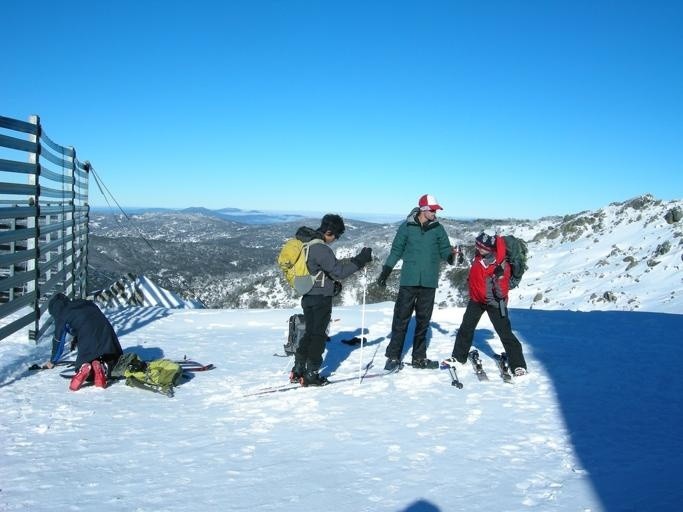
[475,230,496,252]
[419,194,443,211]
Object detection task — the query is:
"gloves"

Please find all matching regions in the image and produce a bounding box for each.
[447,253,463,265]
[378,265,392,288]
[494,264,504,278]
[350,248,373,270]
[334,281,342,296]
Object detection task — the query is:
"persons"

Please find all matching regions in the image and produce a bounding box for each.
[288,213,372,387]
[42,291,122,391]
[377,192,463,372]
[444,228,529,376]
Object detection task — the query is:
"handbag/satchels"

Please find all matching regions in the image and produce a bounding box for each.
[284,314,307,356]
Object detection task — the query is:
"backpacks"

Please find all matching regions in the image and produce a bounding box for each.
[124,359,183,398]
[500,235,528,290]
[277,238,329,295]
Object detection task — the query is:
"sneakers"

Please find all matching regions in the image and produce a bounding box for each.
[92,360,107,388]
[515,367,527,376]
[70,363,92,390]
[384,358,400,371]
[412,359,439,369]
[290,365,328,387]
[442,358,462,368]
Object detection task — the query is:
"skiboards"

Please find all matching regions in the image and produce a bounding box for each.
[59,360,214,381]
[243,363,397,396]
[467,346,511,382]
[385,357,438,373]
